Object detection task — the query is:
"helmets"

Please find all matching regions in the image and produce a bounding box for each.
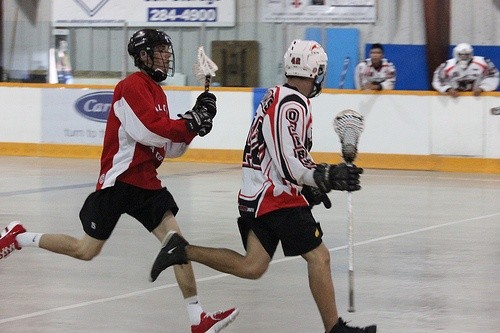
[454,42,474,55]
[283,37,328,80]
[127,28,177,83]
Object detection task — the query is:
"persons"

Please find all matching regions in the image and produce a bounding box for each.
[355,43,396,90]
[0,29,240,333]
[149,39,377,333]
[432,43,500,97]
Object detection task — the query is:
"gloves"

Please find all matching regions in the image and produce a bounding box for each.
[301,184,332,210]
[201,118,213,134]
[313,162,364,193]
[178,92,217,131]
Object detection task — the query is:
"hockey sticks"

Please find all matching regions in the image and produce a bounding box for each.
[193,45,219,137]
[338,56,350,89]
[334,109,365,313]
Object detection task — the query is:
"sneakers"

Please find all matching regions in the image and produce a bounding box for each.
[324,316,377,333]
[148,230,190,283]
[190,307,240,333]
[0,221,28,261]
